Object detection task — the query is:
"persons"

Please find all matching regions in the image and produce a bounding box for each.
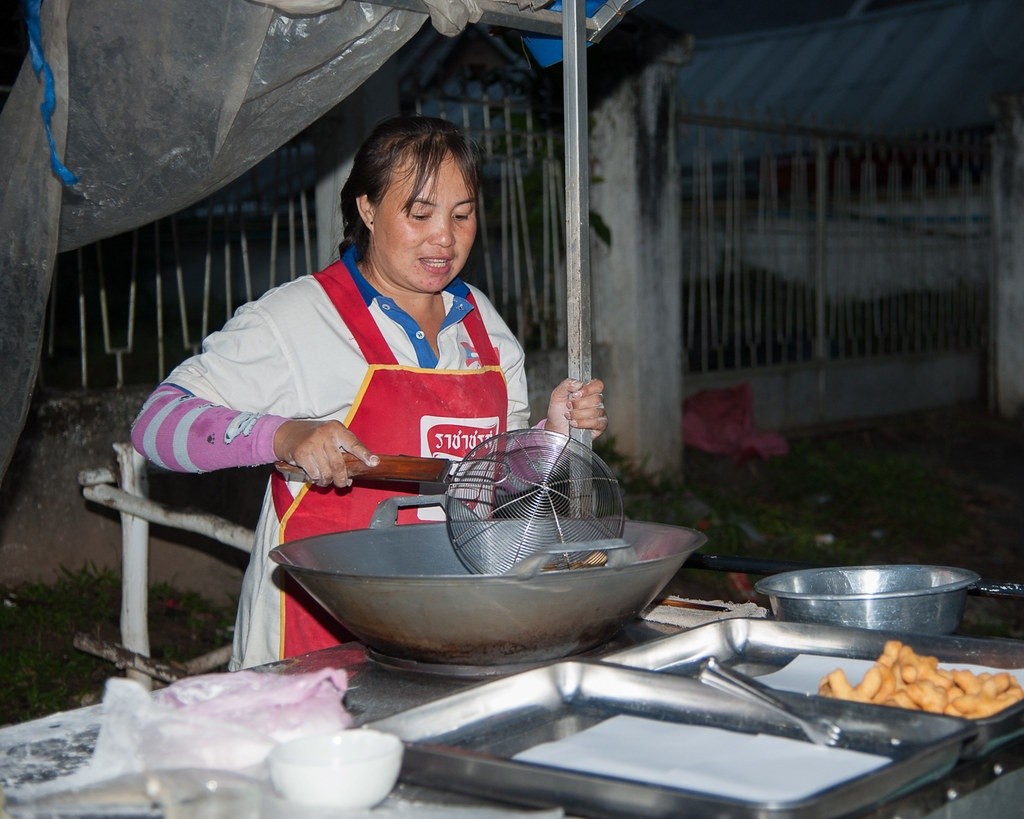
[129,114,609,675]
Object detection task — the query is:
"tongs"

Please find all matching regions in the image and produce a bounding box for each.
[698,658,850,752]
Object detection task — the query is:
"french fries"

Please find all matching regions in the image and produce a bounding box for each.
[819,640,1024,719]
[545,551,608,569]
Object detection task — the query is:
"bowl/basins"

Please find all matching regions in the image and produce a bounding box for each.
[267,730,402,807]
[755,565,981,635]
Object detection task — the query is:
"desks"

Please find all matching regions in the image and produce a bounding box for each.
[1,621,1024,819]
